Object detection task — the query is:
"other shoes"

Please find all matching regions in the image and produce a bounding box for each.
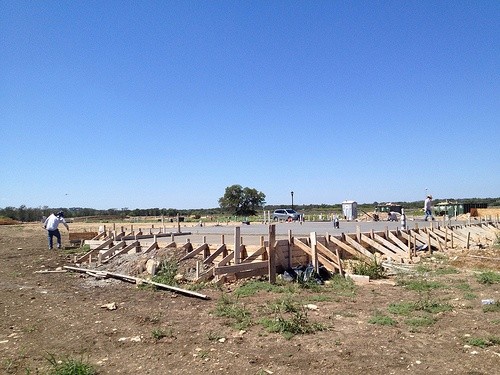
[57,246,61,249]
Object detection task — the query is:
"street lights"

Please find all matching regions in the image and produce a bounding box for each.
[291,191,294,209]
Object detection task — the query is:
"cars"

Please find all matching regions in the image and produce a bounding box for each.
[272,209,301,222]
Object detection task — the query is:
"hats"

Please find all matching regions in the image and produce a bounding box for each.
[428,195,432,199]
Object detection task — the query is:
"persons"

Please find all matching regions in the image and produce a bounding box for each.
[44,210,69,249]
[388,212,398,222]
[425,195,434,221]
